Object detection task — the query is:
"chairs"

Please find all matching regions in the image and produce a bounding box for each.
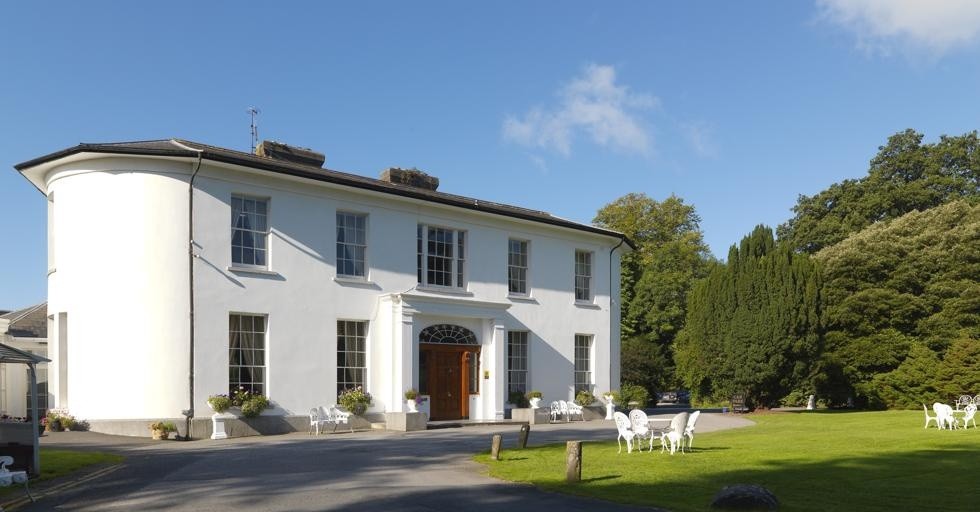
[310,406,354,436]
[550,400,585,423]
[613,409,701,455]
[923,395,980,431]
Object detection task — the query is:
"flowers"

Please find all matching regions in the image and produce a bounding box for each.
[339,385,372,415]
[207,385,268,417]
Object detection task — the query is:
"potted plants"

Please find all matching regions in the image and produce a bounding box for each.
[405,390,418,413]
[602,390,618,403]
[45,410,76,432]
[528,391,542,409]
[148,420,178,440]
